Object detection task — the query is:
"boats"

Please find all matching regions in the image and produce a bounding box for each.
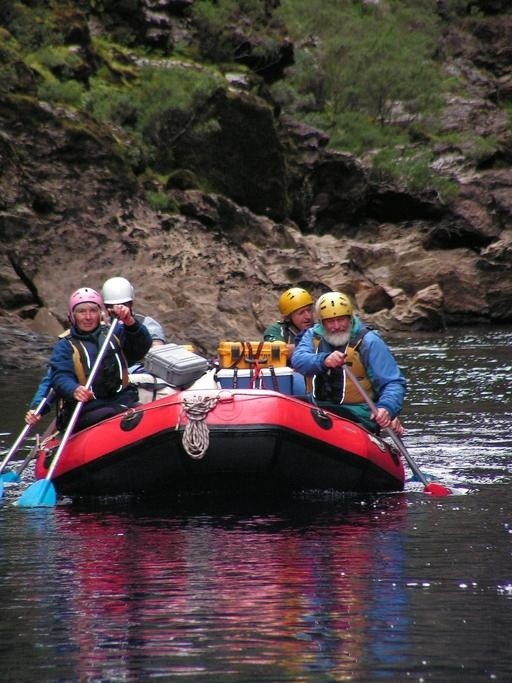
[34,388,405,509]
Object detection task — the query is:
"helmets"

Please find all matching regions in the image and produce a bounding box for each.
[68,287,104,323]
[316,291,353,319]
[102,276,137,308]
[278,288,314,317]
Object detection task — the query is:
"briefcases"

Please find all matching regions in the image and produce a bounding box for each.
[142,343,208,385]
[218,341,287,368]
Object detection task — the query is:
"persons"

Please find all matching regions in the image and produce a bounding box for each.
[263,285,404,442]
[49,285,152,436]
[290,291,407,433]
[24,274,163,422]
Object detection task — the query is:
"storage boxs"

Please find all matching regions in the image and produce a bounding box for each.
[216,367,306,397]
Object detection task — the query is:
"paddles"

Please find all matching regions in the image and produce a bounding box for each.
[17,315,118,517]
[343,359,451,496]
[0,417,59,482]
